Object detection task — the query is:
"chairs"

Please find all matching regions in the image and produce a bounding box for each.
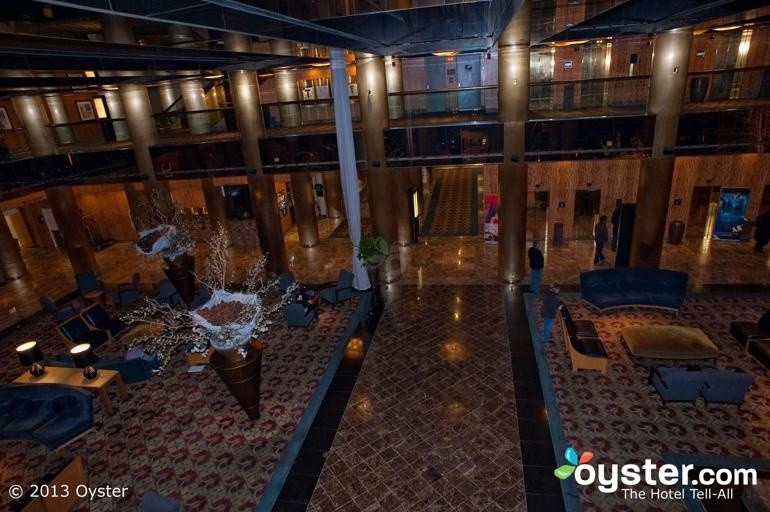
[40,269,219,368]
[646,363,753,410]
[556,300,609,374]
[728,308,770,377]
[274,269,355,329]
[8,453,87,512]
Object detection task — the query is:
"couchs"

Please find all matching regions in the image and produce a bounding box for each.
[47,353,160,386]
[0,383,97,458]
[579,267,689,317]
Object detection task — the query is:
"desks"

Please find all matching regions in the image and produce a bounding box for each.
[11,365,129,414]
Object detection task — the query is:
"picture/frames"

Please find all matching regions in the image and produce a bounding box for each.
[0,106,14,132]
[74,100,97,122]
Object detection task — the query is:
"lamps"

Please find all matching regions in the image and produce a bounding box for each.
[16,341,44,378]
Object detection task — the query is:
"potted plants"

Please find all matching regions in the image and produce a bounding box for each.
[357,236,389,305]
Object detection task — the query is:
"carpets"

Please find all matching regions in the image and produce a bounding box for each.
[0,286,373,512]
[522,290,770,512]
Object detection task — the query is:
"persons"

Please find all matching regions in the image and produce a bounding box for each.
[296,294,322,321]
[752,210,770,253]
[268,115,281,129]
[539,281,565,345]
[528,240,544,297]
[593,215,608,265]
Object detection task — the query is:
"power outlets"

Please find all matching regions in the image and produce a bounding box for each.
[70,342,99,379]
